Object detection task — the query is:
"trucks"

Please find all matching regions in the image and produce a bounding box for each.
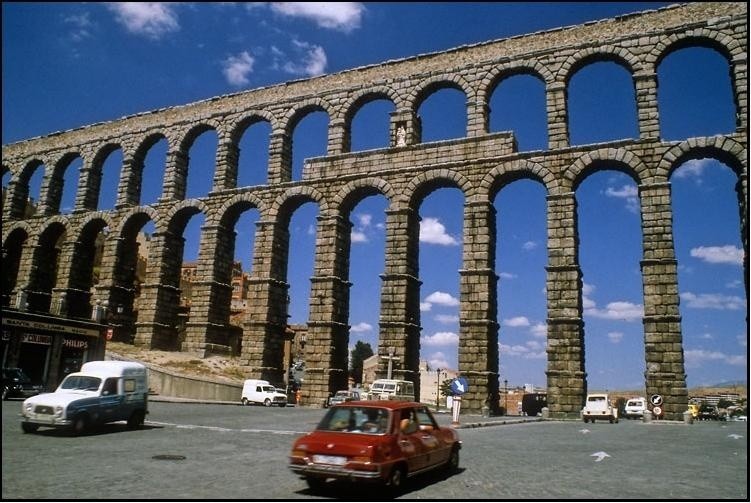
[521,392,546,415]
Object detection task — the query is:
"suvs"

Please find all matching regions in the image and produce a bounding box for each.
[241,379,287,407]
[22,362,147,435]
[328,390,359,407]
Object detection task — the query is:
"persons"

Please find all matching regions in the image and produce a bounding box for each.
[295,387,304,407]
[396,124,406,147]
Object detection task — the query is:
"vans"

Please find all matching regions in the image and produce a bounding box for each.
[366,378,414,402]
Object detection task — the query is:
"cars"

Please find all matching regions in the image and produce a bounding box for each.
[1,367,42,400]
[581,394,618,423]
[624,398,647,419]
[687,404,729,421]
[288,400,462,492]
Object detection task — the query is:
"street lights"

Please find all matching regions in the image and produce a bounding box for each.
[429,353,448,408]
[503,379,508,413]
